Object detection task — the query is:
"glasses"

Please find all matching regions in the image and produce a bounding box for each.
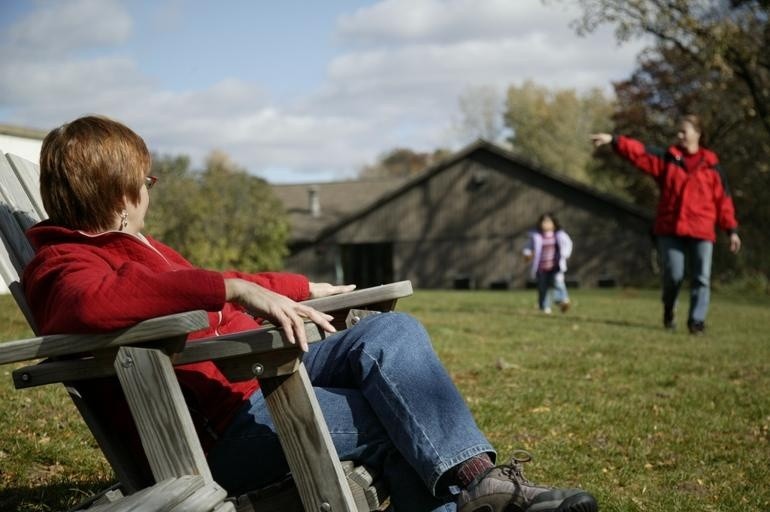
[144,173,160,191]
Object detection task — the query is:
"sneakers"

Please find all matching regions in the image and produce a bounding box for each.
[560,300,571,312]
[456,450,597,511]
[686,319,704,336]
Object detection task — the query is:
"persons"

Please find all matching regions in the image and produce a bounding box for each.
[521,210,574,316]
[589,114,742,335]
[21,116,597,512]
